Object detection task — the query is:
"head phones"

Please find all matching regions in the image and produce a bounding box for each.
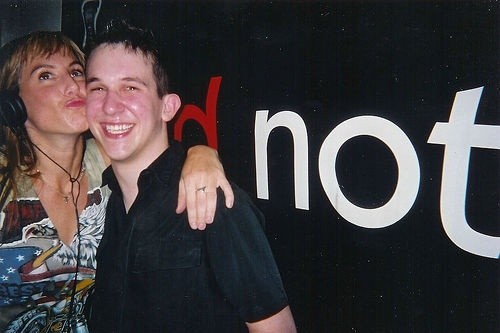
[0,81,27,128]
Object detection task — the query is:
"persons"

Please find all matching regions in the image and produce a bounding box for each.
[0,32,233,333]
[86,24,298,333]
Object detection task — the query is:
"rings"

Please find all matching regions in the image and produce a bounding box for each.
[197,186,206,192]
